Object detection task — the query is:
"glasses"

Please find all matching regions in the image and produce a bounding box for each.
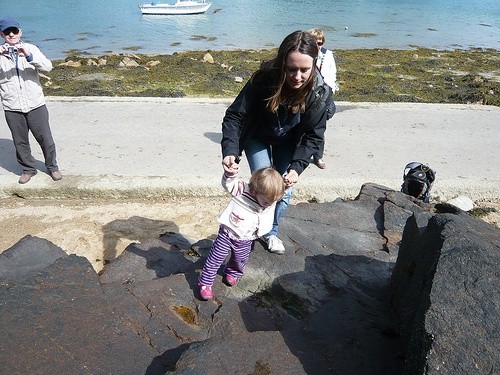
[2,28,19,36]
[318,42,323,46]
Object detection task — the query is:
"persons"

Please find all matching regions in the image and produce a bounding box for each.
[221,29,327,254]
[305,28,336,168]
[198,163,297,299]
[1,18,62,184]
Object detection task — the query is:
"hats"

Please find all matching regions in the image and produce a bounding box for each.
[0,18,20,31]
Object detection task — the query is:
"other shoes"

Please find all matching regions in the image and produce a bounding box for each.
[314,157,326,169]
[198,282,213,300]
[18,169,37,183]
[226,275,237,286]
[50,171,62,181]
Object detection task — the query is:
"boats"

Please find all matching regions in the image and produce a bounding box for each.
[137,0,213,15]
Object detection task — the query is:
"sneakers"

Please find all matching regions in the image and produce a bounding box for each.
[260,234,285,255]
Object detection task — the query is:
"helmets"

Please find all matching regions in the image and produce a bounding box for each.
[400,162,436,203]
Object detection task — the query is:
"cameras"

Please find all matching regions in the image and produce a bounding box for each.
[8,46,19,53]
